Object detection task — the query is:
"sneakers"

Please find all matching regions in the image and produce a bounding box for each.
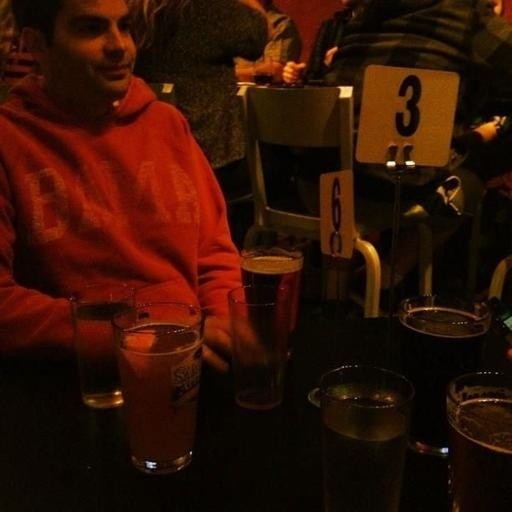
[346,268,407,319]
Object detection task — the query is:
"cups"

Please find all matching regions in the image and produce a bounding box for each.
[238,243,307,336]
[66,281,138,414]
[110,299,206,479]
[396,292,493,460]
[317,362,417,511]
[443,369,512,512]
[227,283,291,413]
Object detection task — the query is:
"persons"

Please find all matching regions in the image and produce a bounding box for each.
[129,2,302,251]
[2,1,266,393]
[282,0,510,299]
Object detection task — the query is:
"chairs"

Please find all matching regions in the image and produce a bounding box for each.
[234,83,434,318]
